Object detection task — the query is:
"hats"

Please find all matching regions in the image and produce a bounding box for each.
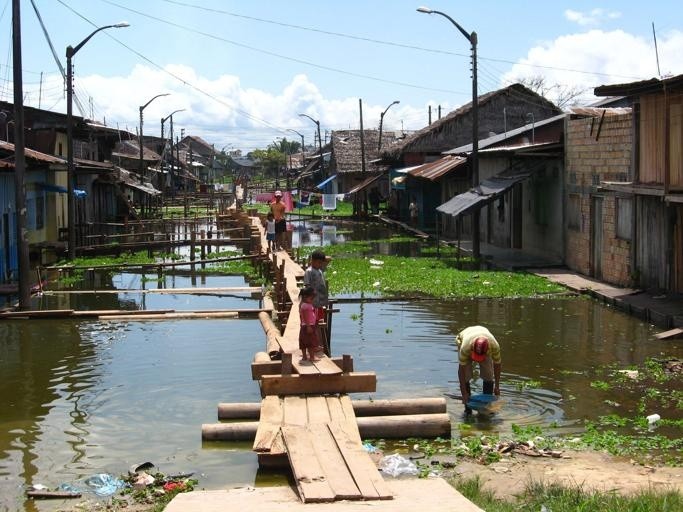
[471,337,488,361]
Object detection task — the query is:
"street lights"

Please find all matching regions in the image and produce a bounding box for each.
[414,6,481,263]
[61,20,131,265]
[298,113,324,180]
[139,93,171,223]
[159,107,185,201]
[377,101,400,149]
[276,128,305,176]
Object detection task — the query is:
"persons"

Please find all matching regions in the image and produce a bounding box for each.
[271,190,286,251]
[455,325,501,415]
[303,250,332,353]
[298,287,321,361]
[264,212,283,252]
